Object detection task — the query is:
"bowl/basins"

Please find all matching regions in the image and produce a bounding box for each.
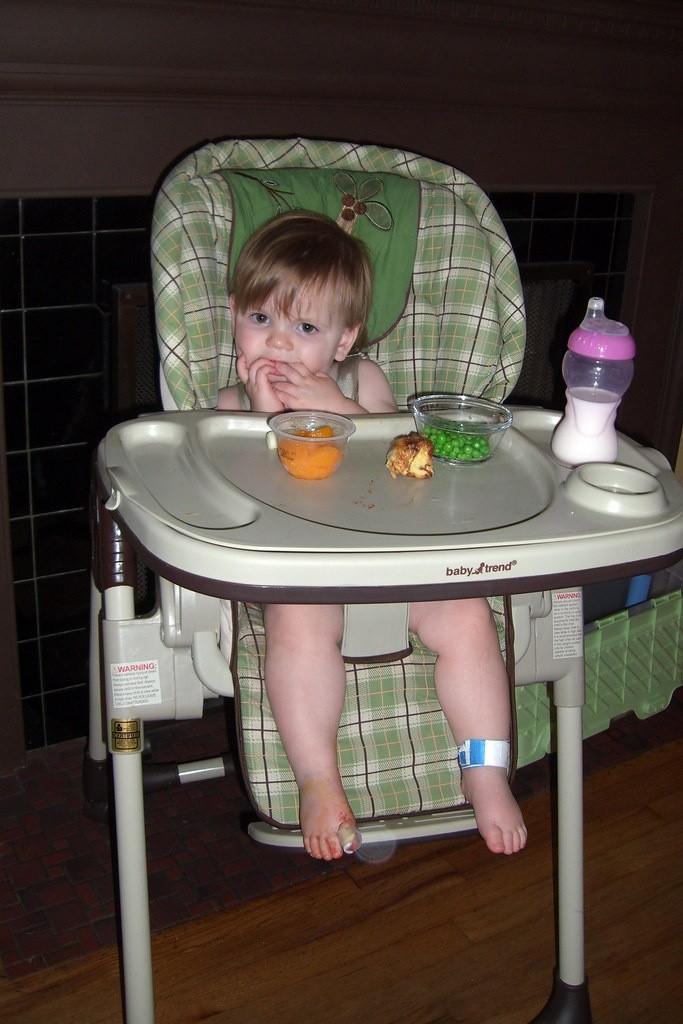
[269,410,356,480]
[410,393,514,466]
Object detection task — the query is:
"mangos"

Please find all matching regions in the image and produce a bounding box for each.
[277,426,343,480]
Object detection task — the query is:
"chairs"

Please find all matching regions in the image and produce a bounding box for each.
[90,140,683,1024]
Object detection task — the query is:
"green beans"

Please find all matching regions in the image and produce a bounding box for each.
[423,419,491,461]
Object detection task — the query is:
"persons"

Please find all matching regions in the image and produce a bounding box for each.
[216,208,528,860]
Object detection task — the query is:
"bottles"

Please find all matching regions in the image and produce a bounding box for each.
[550,297,638,467]
